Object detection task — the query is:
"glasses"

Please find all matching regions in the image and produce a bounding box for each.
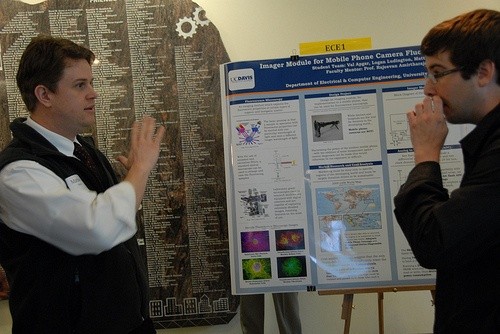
[424,62,481,84]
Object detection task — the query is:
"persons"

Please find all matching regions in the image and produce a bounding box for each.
[0,34,166,334]
[393,8,499,334]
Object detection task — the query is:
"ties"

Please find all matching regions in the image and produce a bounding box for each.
[72,142,104,187]
